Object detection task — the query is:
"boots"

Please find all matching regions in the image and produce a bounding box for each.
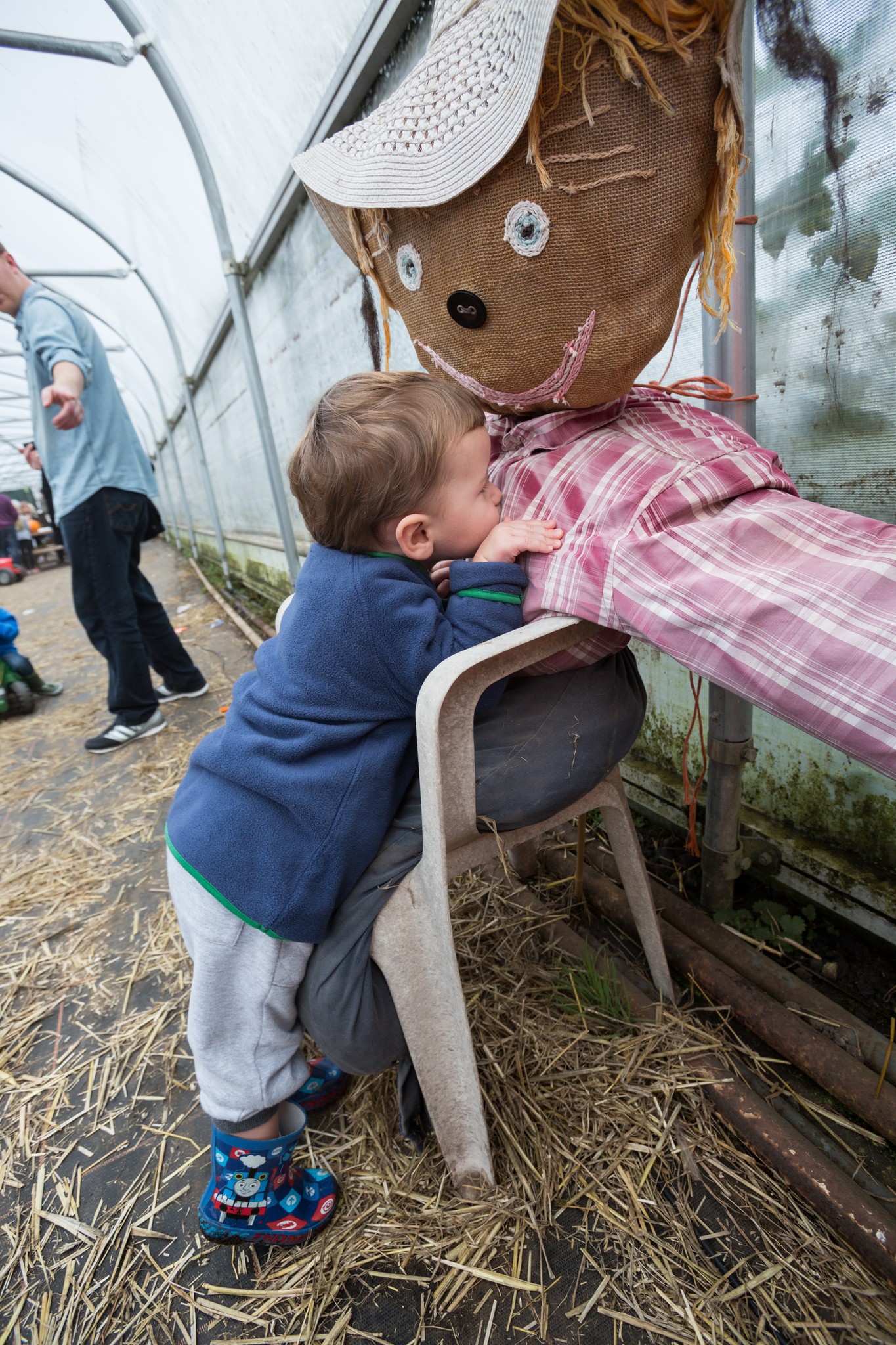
[274,1036,351,1118]
[193,1096,339,1248]
[25,672,64,696]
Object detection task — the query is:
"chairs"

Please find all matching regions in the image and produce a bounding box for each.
[362,608,678,1186]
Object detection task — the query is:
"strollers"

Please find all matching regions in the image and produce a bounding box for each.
[0,659,35,719]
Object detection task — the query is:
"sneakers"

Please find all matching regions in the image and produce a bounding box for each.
[83,704,168,754]
[151,678,210,705]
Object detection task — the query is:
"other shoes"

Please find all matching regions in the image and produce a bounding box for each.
[25,568,40,575]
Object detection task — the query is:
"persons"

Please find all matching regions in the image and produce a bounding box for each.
[164,370,566,1238]
[0,487,70,574]
[288,0,896,1138]
[0,607,65,697]
[0,240,210,754]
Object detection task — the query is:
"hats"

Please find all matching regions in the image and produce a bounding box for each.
[282,1,565,216]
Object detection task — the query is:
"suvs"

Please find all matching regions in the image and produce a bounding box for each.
[0,556,27,588]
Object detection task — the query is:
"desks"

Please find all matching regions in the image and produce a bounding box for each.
[28,524,54,547]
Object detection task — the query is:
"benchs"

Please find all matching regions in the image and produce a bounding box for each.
[31,543,65,570]
